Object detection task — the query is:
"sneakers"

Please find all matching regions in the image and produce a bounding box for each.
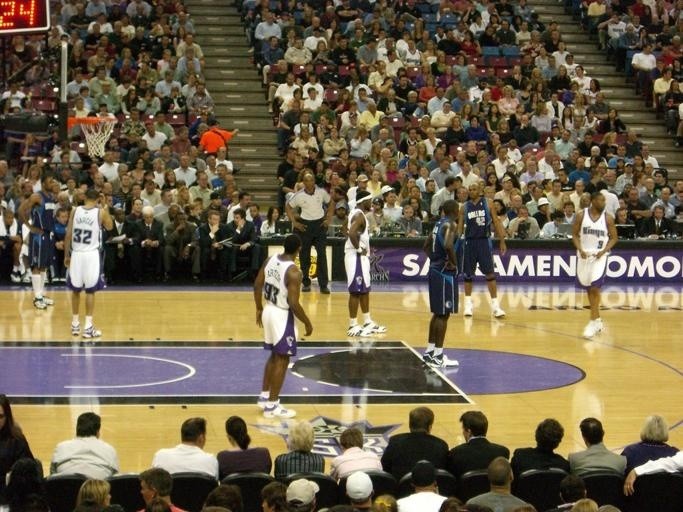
[584,317,603,338]
[464,301,473,316]
[320,286,330,294]
[100,273,106,288]
[346,326,369,337]
[364,322,387,334]
[302,284,310,292]
[423,349,460,368]
[492,306,506,318]
[33,295,54,309]
[71,322,80,335]
[10,271,67,286]
[83,329,101,338]
[257,395,296,418]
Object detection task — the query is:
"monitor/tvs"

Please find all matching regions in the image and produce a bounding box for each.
[275,220,292,235]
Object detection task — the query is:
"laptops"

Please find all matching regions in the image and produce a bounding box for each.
[615,224,636,239]
[671,219,683,237]
[557,224,574,236]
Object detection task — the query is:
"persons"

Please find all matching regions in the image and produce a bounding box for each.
[0,1,683,294]
[421,198,461,367]
[2,391,681,511]
[62,189,114,341]
[253,235,314,418]
[18,175,57,310]
[344,190,388,337]
[572,191,619,341]
[457,182,508,319]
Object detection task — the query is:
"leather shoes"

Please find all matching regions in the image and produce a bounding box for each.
[109,269,226,287]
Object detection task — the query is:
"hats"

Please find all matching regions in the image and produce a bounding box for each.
[358,175,369,182]
[624,157,634,166]
[285,479,319,506]
[210,193,221,199]
[344,471,372,499]
[336,201,347,210]
[207,119,220,126]
[380,185,396,195]
[538,197,549,206]
[412,459,437,487]
[502,177,513,182]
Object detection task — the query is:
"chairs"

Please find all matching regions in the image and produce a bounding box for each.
[446,45,527,81]
[23,85,203,153]
[422,10,461,34]
[291,63,351,77]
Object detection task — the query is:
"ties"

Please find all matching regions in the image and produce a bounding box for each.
[657,220,659,231]
[146,226,150,238]
[117,225,124,259]
